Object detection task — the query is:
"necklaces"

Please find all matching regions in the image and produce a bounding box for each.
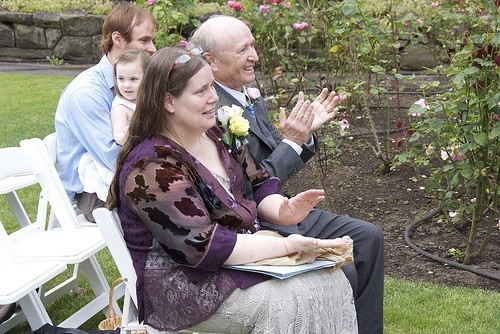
[205,168,230,187]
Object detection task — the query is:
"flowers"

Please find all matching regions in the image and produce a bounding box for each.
[245,87,261,106]
[217,104,250,149]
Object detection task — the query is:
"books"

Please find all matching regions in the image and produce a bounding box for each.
[221,260,336,280]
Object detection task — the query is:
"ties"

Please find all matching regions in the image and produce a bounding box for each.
[245,96,256,122]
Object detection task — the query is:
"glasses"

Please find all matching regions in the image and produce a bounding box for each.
[171,47,203,72]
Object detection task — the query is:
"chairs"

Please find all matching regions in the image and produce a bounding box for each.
[0,132,138,334]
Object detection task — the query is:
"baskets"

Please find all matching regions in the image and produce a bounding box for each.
[97,278,146,334]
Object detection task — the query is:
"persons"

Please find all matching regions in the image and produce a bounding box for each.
[111,48,152,146]
[105,47,358,334]
[187,14,384,334]
[55,3,159,222]
